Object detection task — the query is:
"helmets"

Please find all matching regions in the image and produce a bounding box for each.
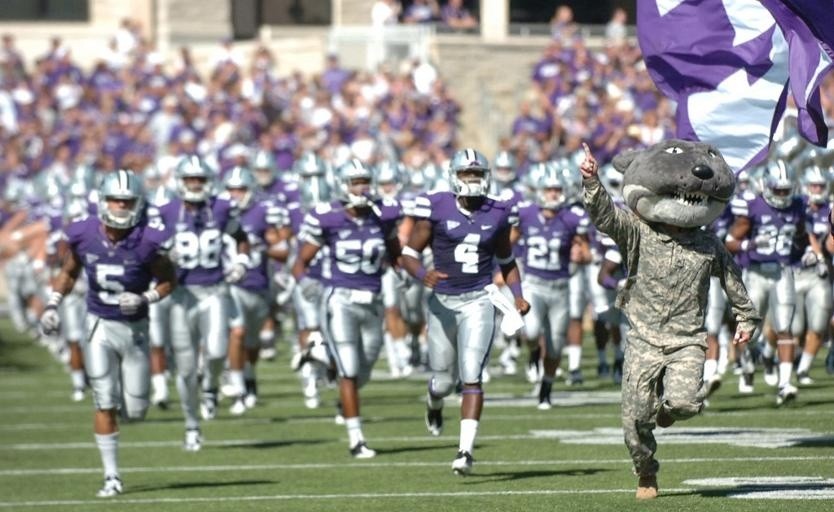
[448,149,490,200]
[98,168,145,230]
[10,156,260,212]
[493,151,623,210]
[734,136,834,204]
[296,152,438,207]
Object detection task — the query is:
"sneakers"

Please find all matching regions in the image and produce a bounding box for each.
[95,480,122,498]
[565,357,832,405]
[350,441,375,459]
[654,403,676,427]
[182,426,202,452]
[260,339,554,412]
[71,381,257,419]
[452,450,471,477]
[425,393,444,436]
[636,473,658,499]
